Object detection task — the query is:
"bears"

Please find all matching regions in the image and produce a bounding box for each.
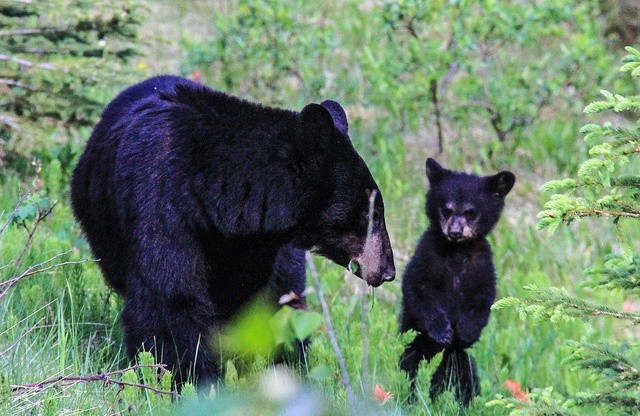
[396,157,517,409]
[69,74,396,400]
